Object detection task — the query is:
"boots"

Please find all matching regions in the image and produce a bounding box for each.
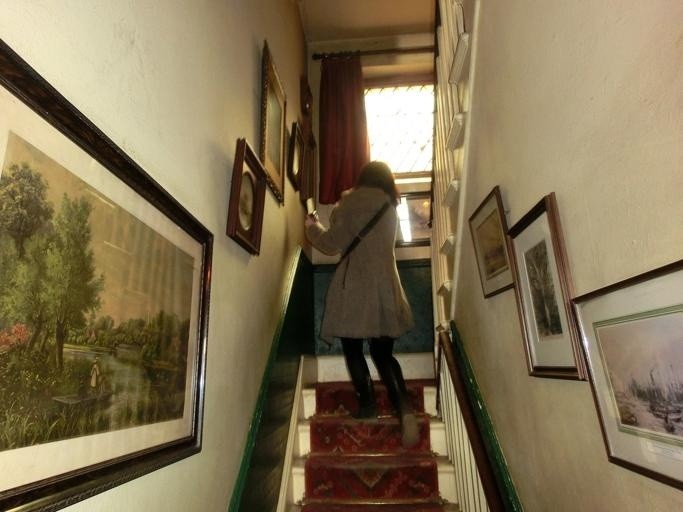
[380,372,419,448]
[348,360,378,419]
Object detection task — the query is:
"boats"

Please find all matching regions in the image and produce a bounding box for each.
[52,388,113,414]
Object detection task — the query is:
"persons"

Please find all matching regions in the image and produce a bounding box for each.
[305,163,421,449]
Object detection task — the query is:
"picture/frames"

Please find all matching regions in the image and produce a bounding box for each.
[0,38,214,512]
[288,77,318,214]
[569,259,683,491]
[227,138,267,256]
[260,39,286,206]
[468,186,516,298]
[392,190,434,248]
[505,191,589,382]
